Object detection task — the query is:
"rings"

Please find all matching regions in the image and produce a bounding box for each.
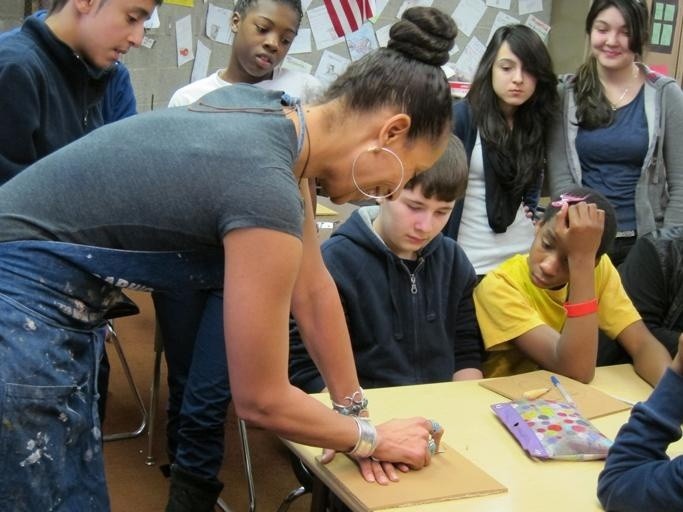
[430,419,440,435]
[428,434,436,454]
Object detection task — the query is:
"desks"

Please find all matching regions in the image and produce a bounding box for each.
[277,362,683,512]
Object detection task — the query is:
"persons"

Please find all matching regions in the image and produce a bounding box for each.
[597,332,683,512]
[472,186,671,389]
[286,135,484,391]
[161,1,301,510]
[448,23,559,278]
[597,224,683,365]
[0,8,459,511]
[543,1,683,269]
[0,1,155,182]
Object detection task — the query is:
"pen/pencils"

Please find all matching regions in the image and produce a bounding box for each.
[551,375,576,409]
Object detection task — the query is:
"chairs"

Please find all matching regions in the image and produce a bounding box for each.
[94,290,150,442]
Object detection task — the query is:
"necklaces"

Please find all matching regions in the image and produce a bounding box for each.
[600,64,639,111]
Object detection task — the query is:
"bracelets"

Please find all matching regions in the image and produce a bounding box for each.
[563,298,598,317]
[331,386,369,417]
[345,415,377,458]
